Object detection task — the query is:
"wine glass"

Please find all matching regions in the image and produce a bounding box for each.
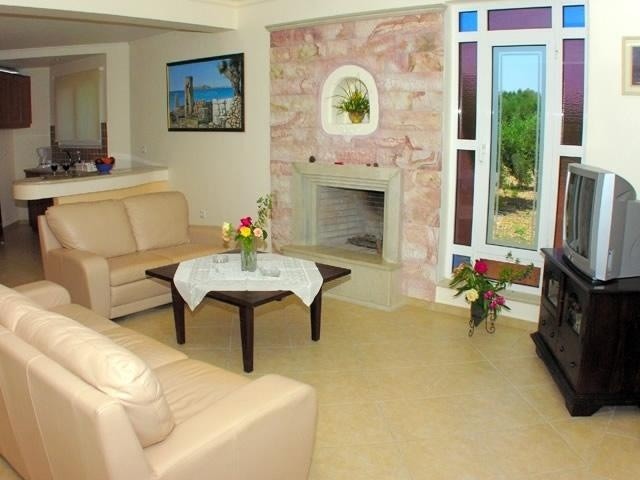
[37,147,52,168]
[63,163,71,177]
[51,164,57,177]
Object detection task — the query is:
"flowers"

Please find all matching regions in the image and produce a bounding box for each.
[448,251,534,326]
[326,72,370,118]
[221,192,275,266]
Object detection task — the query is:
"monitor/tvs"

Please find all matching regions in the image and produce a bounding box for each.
[562,162,640,285]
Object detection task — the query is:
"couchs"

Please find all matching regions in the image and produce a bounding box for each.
[38,180,266,320]
[0,280,318,480]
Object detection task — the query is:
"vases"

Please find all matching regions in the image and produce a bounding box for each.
[349,111,364,123]
[238,240,257,272]
[471,296,488,327]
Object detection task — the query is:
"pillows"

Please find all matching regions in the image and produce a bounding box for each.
[0,284,174,449]
[46,190,190,258]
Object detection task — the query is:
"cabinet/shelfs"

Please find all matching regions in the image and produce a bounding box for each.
[0,72,32,128]
[530,247,640,417]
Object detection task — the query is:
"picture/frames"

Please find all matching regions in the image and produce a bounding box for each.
[622,35,640,95]
[165,53,245,132]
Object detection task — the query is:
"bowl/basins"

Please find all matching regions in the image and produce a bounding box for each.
[95,163,113,173]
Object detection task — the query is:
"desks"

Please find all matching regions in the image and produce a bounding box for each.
[12,166,168,232]
